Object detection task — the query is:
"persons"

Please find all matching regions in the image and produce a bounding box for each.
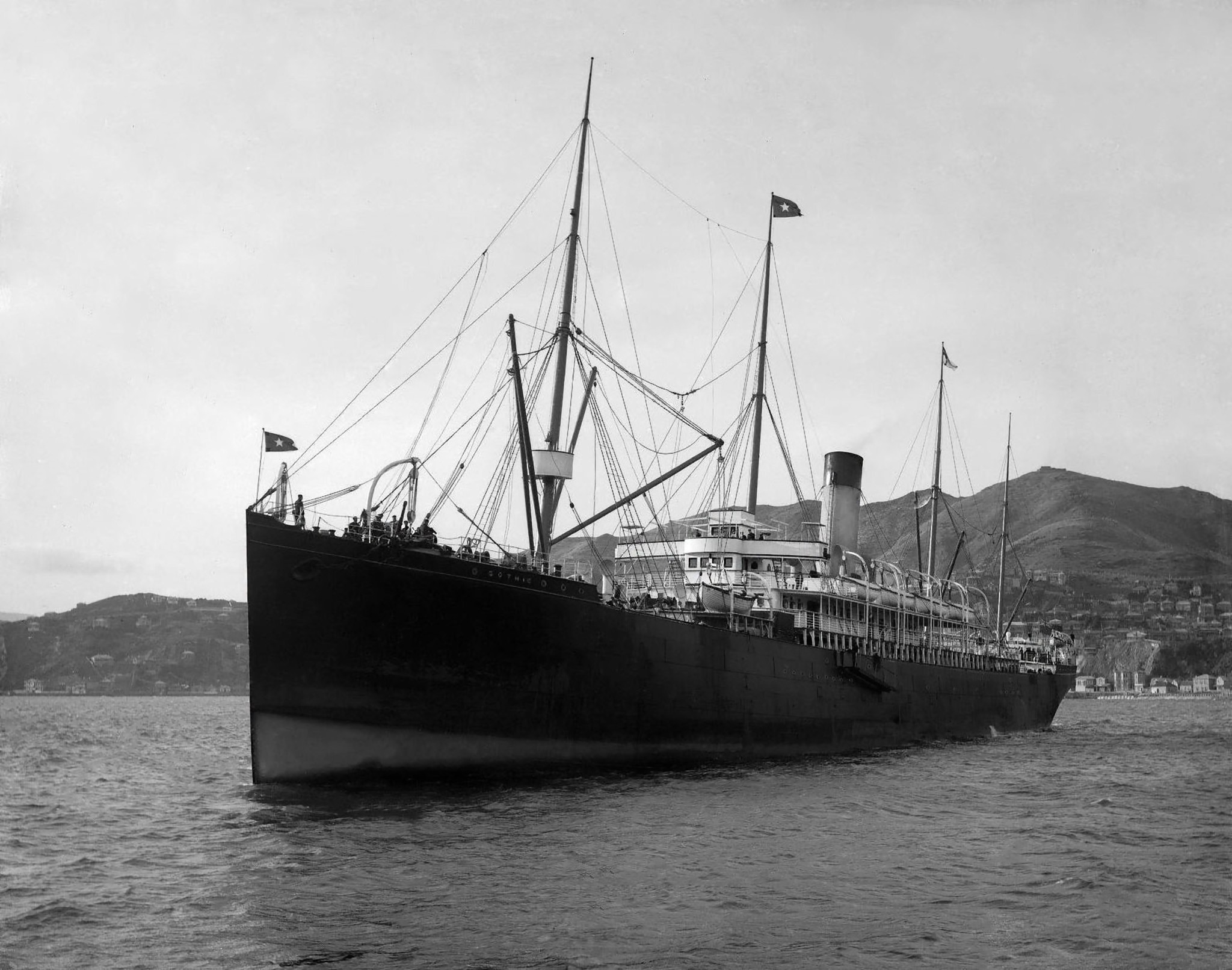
[1019,645,1073,665]
[292,495,804,611]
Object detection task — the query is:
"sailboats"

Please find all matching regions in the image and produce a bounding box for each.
[247,54,1078,789]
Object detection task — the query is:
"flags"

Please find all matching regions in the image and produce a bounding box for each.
[941,346,958,371]
[771,195,804,218]
[264,433,298,453]
